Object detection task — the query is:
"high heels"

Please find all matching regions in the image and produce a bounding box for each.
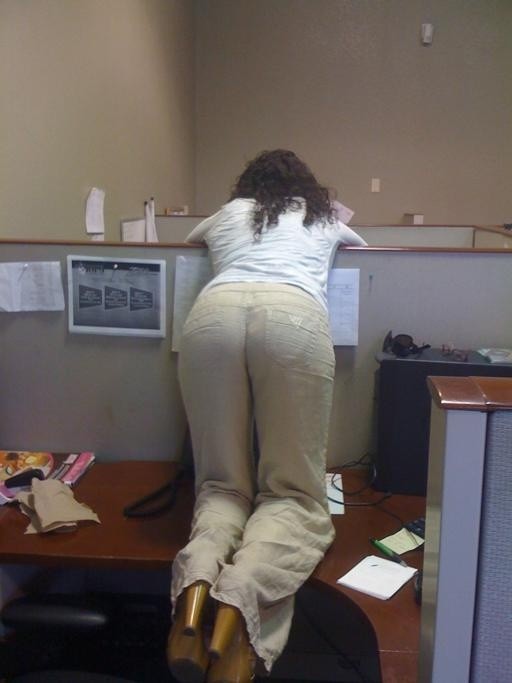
[166,581,212,682]
[208,602,257,683]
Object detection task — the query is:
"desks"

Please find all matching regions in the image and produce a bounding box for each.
[0,452,425,683]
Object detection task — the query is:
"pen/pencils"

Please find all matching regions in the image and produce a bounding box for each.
[367,535,410,567]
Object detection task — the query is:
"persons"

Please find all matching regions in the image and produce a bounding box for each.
[168,148,366,682]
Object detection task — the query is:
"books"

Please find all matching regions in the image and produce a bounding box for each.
[0,449,96,505]
[335,555,419,600]
[382,528,423,555]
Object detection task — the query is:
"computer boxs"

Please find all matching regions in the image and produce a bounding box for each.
[370,347,512,497]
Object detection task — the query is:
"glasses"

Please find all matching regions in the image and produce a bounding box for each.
[382,330,431,356]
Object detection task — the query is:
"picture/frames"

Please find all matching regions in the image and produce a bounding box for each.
[65,254,170,339]
